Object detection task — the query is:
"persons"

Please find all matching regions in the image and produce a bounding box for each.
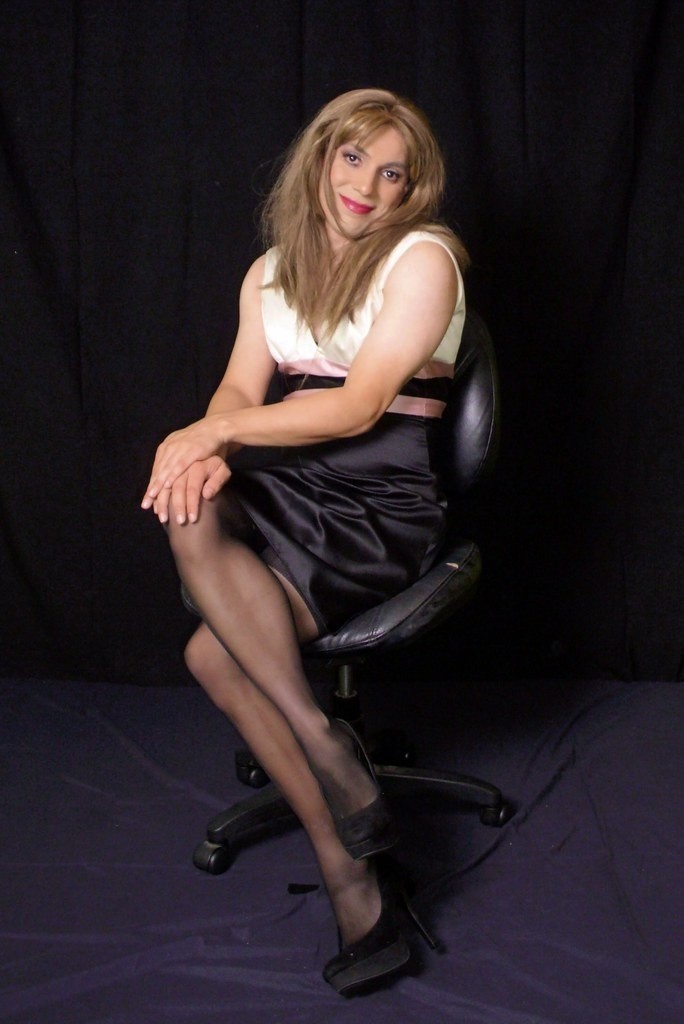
[139,89,480,991]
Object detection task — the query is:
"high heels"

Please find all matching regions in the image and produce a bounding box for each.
[323,857,439,995]
[320,718,399,861]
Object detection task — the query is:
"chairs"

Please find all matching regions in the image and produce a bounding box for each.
[180,293,512,875]
